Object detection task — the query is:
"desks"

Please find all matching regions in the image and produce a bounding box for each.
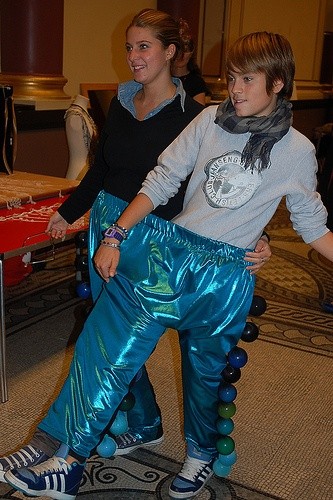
[0,194,91,403]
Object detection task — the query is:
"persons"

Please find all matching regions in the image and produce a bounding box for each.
[0,8,273,484]
[170,34,211,106]
[3,31,333,500]
[63,95,95,181]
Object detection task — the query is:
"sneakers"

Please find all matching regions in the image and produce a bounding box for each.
[0,443,85,500]
[169,440,219,499]
[111,416,164,455]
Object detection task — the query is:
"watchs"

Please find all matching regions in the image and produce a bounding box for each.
[101,227,124,244]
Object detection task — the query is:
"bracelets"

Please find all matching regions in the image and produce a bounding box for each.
[100,239,121,252]
[108,223,129,240]
